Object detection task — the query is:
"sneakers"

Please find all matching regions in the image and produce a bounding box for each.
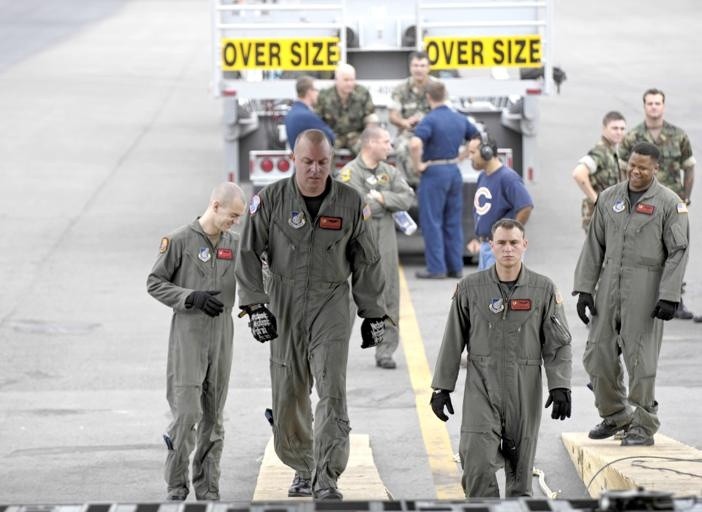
[589,420,630,439]
[166,495,186,502]
[620,426,654,446]
[415,270,448,279]
[379,358,395,368]
[448,271,465,278]
[674,302,693,319]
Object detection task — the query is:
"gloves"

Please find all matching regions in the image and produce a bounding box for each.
[545,388,571,420]
[238,303,278,343]
[577,292,596,324]
[430,388,454,421]
[651,299,679,321]
[185,291,224,318]
[361,318,385,349]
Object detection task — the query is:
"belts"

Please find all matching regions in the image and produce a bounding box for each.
[431,159,454,164]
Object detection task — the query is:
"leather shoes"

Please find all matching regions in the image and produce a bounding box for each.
[288,474,313,497]
[314,487,344,499]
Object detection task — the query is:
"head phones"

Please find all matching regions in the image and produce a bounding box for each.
[480,132,494,160]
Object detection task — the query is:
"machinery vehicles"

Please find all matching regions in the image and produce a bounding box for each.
[212,2,566,266]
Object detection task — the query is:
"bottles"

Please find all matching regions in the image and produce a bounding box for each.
[391,209,417,236]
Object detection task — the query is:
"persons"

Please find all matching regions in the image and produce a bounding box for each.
[333,127,418,370]
[314,63,375,153]
[570,142,689,447]
[407,80,481,280]
[622,89,696,321]
[430,219,572,500]
[146,181,250,503]
[232,128,389,500]
[285,75,337,154]
[389,50,450,187]
[573,110,626,237]
[468,131,535,274]
